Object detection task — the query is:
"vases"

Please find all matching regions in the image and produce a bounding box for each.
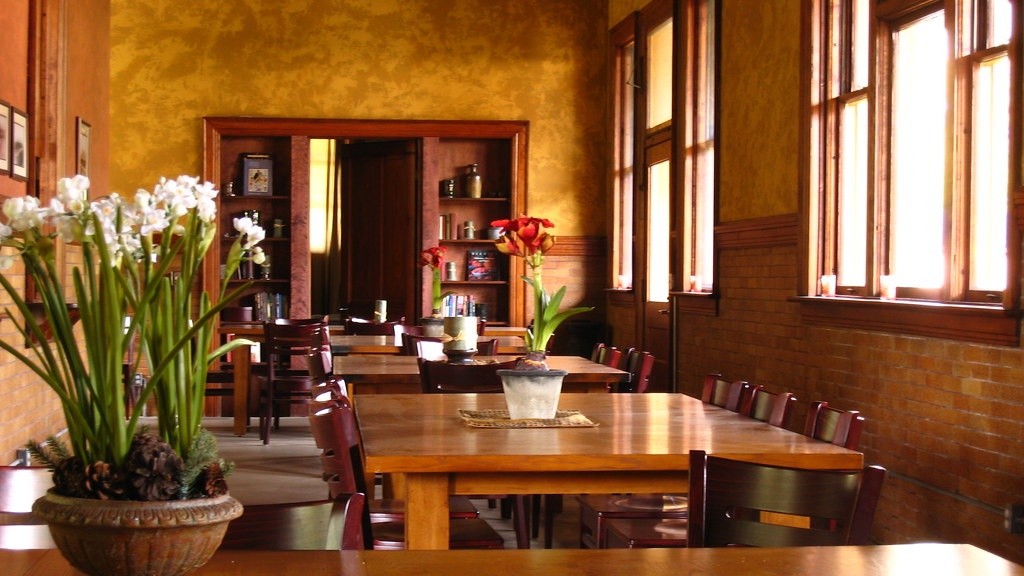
[496,369,568,420]
[32,486,244,576]
[421,318,444,337]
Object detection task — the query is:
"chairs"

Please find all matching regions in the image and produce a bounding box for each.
[187,317,885,551]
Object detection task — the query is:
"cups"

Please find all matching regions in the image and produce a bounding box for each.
[878,275,897,299]
[821,274,836,294]
[689,275,702,292]
[618,274,629,288]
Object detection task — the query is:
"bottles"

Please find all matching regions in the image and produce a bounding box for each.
[464,163,482,199]
[444,179,455,197]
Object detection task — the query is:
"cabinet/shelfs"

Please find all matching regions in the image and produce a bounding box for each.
[218,194,289,325]
[437,196,518,324]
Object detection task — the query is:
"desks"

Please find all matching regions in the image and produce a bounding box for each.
[328,334,527,357]
[220,325,529,439]
[0,543,1024,576]
[333,356,630,407]
[352,393,863,549]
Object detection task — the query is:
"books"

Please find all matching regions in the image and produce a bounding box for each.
[443,294,476,316]
[467,250,502,281]
[227,334,232,363]
[236,252,255,280]
[439,213,463,240]
[254,293,288,320]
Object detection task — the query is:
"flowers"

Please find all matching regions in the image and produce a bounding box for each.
[0,173,271,498]
[492,217,596,370]
[420,246,457,318]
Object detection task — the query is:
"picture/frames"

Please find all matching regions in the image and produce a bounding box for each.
[75,117,91,182]
[11,105,30,181]
[0,99,12,175]
[243,158,272,196]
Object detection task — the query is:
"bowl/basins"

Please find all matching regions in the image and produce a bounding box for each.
[487,225,506,240]
